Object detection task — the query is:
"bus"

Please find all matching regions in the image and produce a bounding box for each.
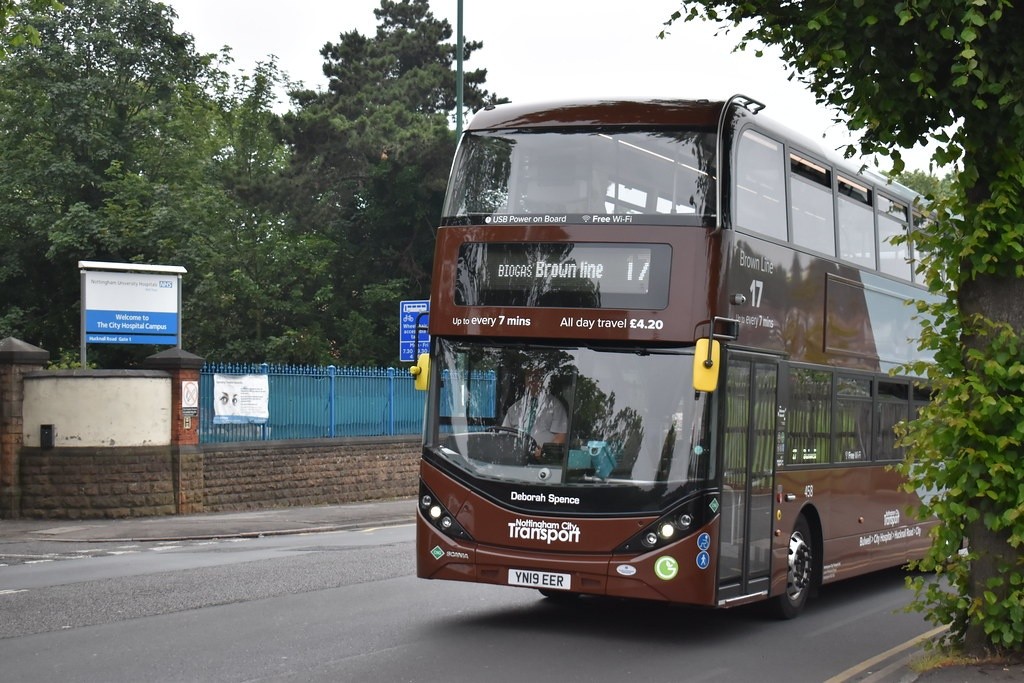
[409,90,966,624]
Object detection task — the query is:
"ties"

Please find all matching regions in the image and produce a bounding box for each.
[527,399,537,436]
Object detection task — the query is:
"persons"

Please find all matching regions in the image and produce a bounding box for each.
[497,362,568,462]
